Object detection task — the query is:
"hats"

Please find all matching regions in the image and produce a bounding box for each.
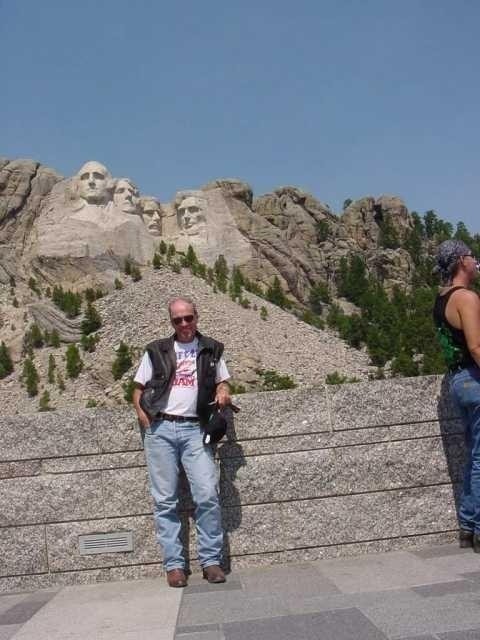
[202,400,228,446]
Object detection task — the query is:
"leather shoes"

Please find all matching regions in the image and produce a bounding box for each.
[202,566,225,584]
[459,529,479,553]
[166,569,187,587]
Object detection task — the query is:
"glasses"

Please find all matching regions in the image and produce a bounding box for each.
[461,252,475,259]
[173,315,194,325]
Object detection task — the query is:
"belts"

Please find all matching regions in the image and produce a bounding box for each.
[157,413,197,422]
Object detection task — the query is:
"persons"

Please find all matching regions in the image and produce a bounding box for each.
[135,194,164,237]
[172,190,209,239]
[429,238,480,553]
[109,176,142,215]
[130,296,234,585]
[68,160,141,230]
